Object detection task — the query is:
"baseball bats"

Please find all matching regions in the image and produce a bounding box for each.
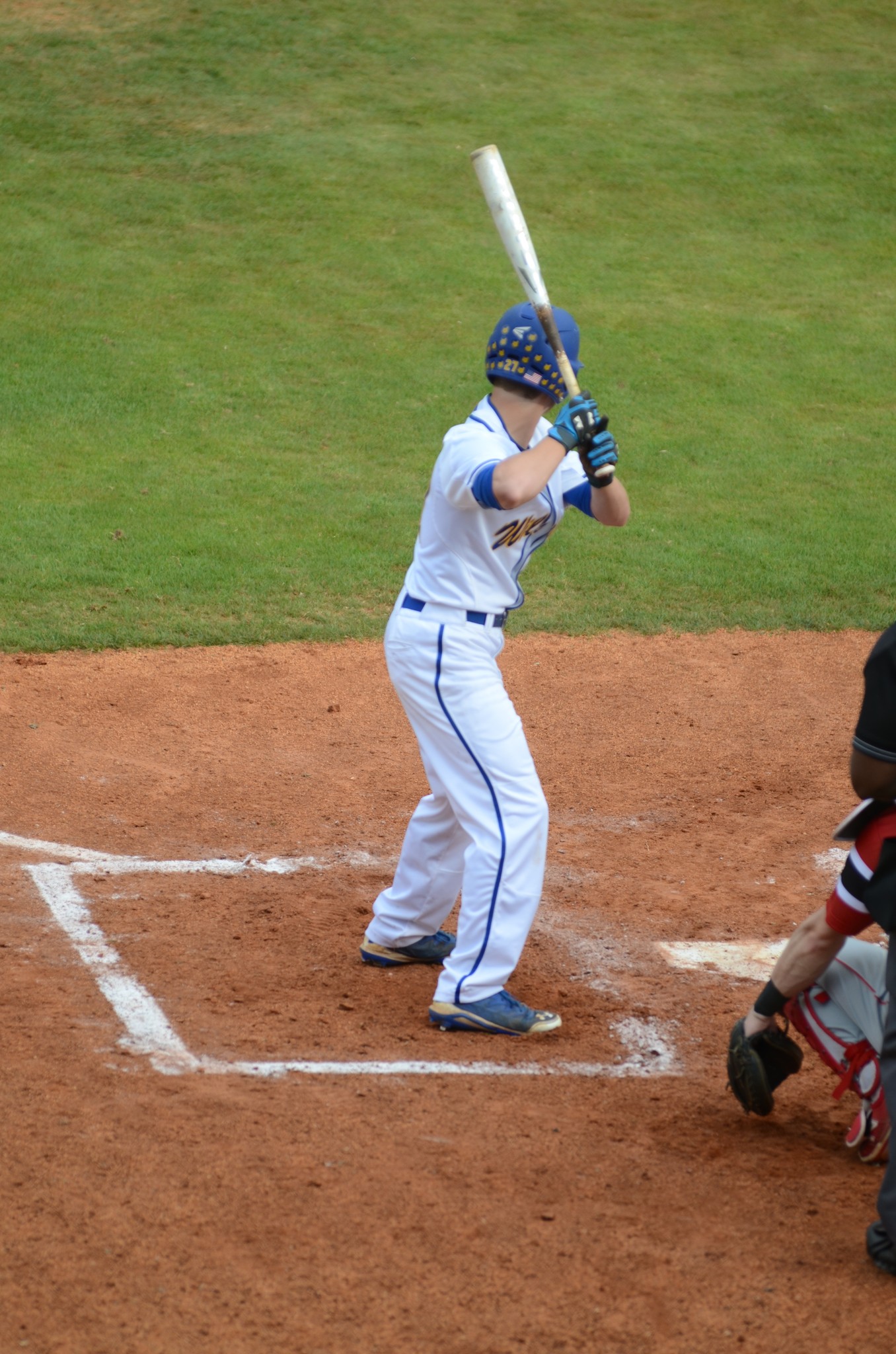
[468,142,616,480]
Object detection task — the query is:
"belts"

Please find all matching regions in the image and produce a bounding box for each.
[402,595,507,628]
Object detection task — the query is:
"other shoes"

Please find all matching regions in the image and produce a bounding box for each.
[865,1219,896,1275]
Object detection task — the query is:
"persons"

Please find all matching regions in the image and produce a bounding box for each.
[725,620,895,1276]
[361,305,631,1037]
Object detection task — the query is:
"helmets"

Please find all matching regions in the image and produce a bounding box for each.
[486,301,584,403]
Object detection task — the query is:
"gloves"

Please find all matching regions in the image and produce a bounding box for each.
[548,388,601,454]
[577,414,619,487]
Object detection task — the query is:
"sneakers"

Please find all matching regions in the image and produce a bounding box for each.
[428,989,562,1036]
[858,1090,893,1163]
[360,928,457,967]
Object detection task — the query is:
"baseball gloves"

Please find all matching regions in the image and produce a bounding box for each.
[724,1014,805,1119]
